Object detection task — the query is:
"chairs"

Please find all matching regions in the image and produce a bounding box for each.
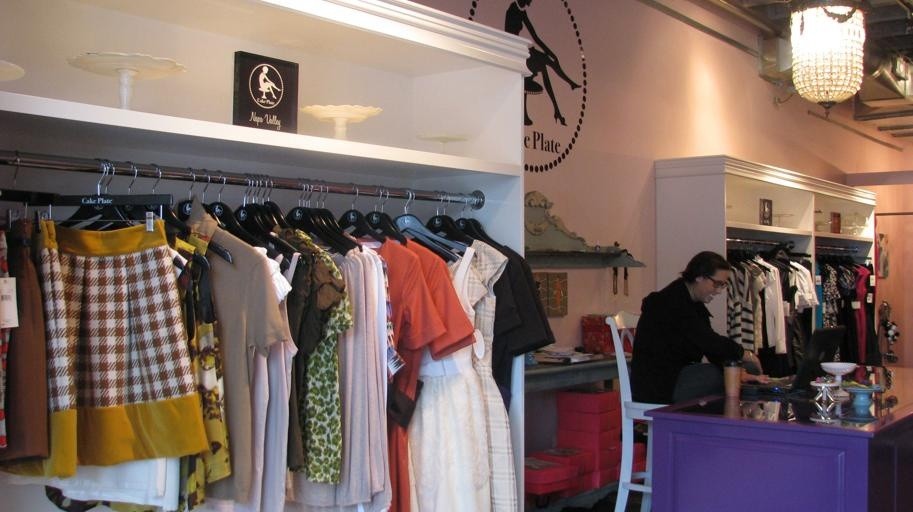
[605,307,674,512]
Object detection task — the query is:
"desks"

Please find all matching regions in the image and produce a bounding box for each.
[523,350,634,512]
[647,361,911,512]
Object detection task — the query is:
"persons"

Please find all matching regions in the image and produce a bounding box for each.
[628,250,769,407]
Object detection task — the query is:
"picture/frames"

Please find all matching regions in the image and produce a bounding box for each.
[231,50,299,135]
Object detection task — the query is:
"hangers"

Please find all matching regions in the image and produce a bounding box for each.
[723,235,867,275]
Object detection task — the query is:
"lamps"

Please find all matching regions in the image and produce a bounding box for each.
[787,0,874,118]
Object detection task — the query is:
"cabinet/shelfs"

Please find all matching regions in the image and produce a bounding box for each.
[649,155,880,355]
[0,0,528,512]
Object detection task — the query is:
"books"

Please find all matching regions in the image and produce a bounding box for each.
[828,210,842,233]
[758,197,773,226]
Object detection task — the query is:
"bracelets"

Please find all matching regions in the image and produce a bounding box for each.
[746,350,753,363]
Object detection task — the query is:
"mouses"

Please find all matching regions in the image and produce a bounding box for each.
[695,400,711,410]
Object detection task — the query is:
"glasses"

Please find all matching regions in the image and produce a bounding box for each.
[706,275,728,288]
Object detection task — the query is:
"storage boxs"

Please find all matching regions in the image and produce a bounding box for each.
[522,379,643,499]
[577,314,634,354]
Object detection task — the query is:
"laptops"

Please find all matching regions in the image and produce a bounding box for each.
[740,326,846,393]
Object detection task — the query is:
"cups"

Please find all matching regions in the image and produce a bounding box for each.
[722,397,741,418]
[719,360,743,399]
